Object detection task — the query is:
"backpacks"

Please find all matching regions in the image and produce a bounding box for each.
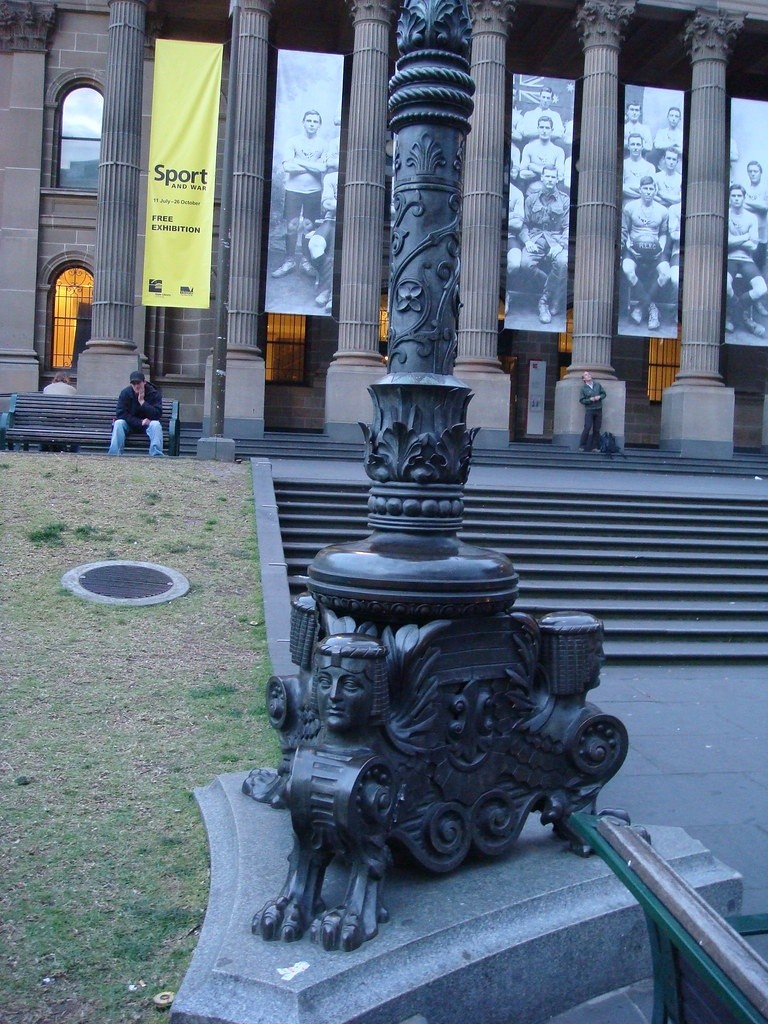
[599,431,619,454]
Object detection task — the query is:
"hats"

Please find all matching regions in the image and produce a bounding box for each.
[129,371,145,383]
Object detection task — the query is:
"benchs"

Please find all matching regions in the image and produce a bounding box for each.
[0,392,181,456]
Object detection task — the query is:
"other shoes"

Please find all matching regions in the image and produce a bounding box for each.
[579,448,585,452]
[299,261,317,276]
[592,449,601,452]
[552,300,560,315]
[755,303,767,316]
[745,320,765,335]
[537,304,551,323]
[726,315,735,332]
[631,307,642,324]
[271,263,296,278]
[316,290,330,305]
[648,304,661,330]
[325,301,332,311]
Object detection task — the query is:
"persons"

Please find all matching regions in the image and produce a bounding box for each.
[107,371,163,456]
[38,372,81,453]
[540,611,605,702]
[504,86,573,322]
[308,635,389,743]
[622,102,683,330]
[726,138,768,336]
[579,372,607,453]
[270,109,341,312]
